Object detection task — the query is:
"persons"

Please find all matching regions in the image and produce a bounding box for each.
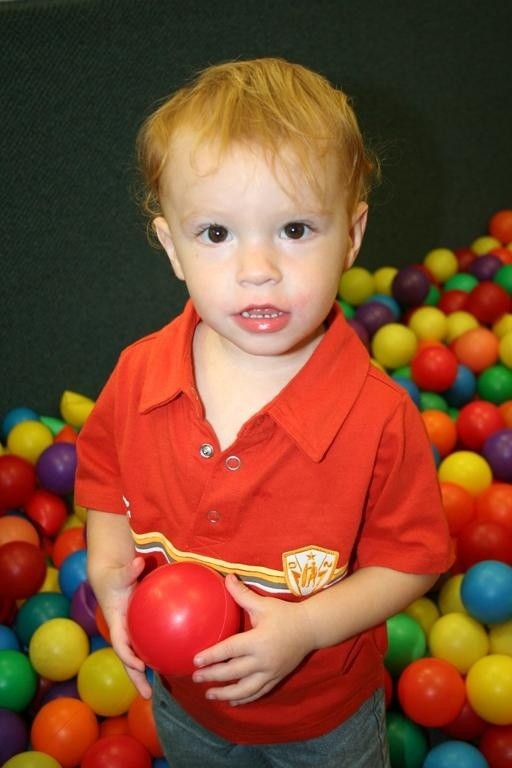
[74,56,458,767]
